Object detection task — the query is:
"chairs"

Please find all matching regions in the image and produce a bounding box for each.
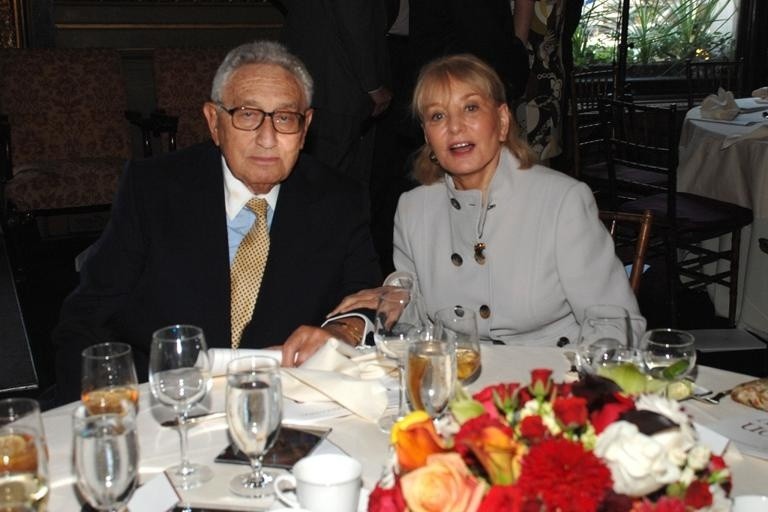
[146,42,245,152]
[683,56,746,107]
[595,206,656,298]
[592,91,757,331]
[568,71,669,213]
[0,42,154,230]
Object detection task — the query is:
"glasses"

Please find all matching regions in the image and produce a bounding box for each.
[221,105,307,135]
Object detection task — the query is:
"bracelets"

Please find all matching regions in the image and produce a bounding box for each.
[328,322,365,346]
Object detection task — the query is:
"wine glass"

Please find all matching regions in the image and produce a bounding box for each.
[222,353,289,498]
[376,287,429,434]
[149,326,215,488]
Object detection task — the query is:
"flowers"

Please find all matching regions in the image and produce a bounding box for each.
[367,371,745,512]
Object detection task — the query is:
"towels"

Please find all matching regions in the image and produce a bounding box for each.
[204,337,393,425]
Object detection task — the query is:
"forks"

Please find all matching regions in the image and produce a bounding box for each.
[690,382,754,404]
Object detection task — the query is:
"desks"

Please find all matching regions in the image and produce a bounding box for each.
[1,251,46,395]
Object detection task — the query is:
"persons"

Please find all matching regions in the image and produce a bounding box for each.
[326,53,648,358]
[45,43,398,406]
[282,0,583,196]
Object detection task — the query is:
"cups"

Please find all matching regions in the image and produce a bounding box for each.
[0,395,50,511]
[431,306,483,380]
[601,346,639,371]
[80,341,145,487]
[272,454,361,511]
[573,305,632,379]
[640,327,695,412]
[70,405,139,512]
[404,337,454,418]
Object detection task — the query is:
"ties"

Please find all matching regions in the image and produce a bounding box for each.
[230,196,271,350]
